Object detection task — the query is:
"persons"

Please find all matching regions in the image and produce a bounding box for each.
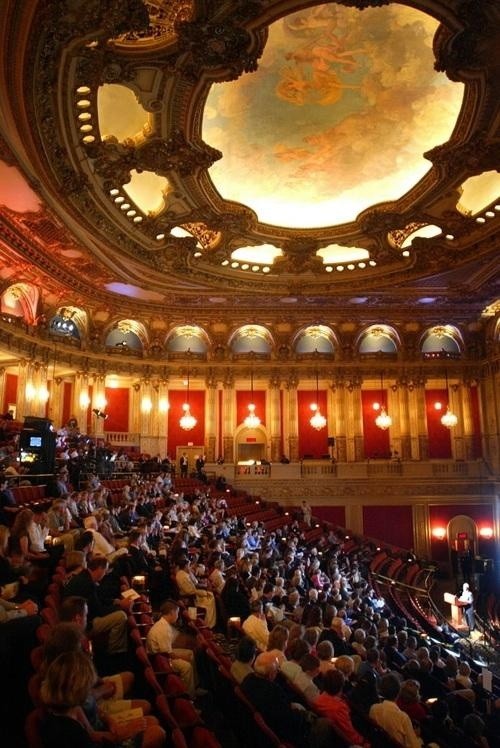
[1,410,497,748]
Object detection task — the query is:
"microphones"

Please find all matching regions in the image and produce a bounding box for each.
[456,591,462,595]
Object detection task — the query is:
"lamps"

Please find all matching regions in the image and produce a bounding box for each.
[26,371,172,421]
[243,350,262,430]
[177,347,198,430]
[373,338,394,430]
[438,339,460,430]
[309,349,328,430]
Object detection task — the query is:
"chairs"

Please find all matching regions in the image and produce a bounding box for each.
[0,473,500,747]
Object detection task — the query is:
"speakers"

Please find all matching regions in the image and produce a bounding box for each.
[328,437,334,446]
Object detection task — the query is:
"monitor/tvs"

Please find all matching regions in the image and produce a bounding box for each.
[30,436,42,447]
